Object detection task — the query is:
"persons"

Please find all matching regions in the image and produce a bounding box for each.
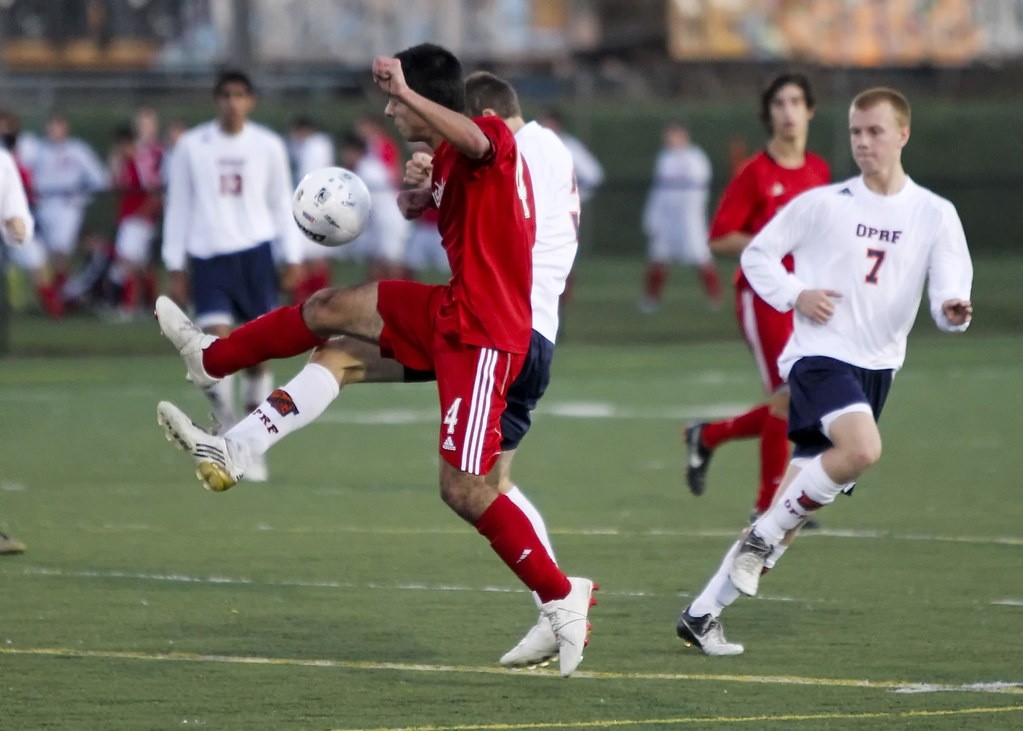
[0,44,726,676]
[684,72,819,532]
[674,87,974,653]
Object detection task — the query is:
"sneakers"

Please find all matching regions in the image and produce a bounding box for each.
[500,615,558,669]
[673,602,744,656]
[155,294,224,389]
[685,423,711,496]
[729,522,785,596]
[748,511,816,528]
[157,401,243,494]
[540,576,598,676]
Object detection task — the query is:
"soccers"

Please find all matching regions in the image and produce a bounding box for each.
[291,166,371,247]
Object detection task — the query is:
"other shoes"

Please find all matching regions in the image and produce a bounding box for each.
[245,456,267,481]
[0,532,26,551]
